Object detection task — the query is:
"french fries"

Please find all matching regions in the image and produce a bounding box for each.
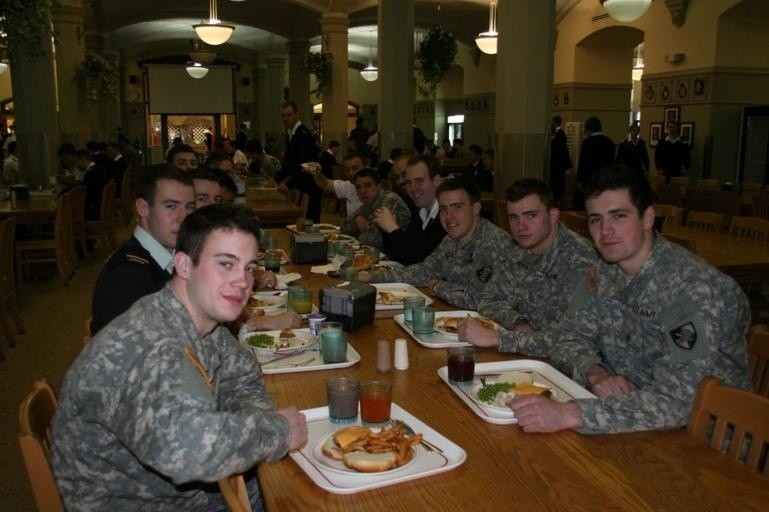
[362,422,423,468]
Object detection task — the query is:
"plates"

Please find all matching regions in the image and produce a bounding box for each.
[248,293,284,312]
[310,433,417,477]
[375,288,413,304]
[243,329,316,350]
[432,322,500,338]
[467,381,559,411]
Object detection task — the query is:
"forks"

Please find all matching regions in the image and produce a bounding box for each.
[262,358,315,370]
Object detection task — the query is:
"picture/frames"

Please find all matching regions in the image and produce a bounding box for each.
[648,122,664,147]
[664,107,680,135]
[680,122,694,149]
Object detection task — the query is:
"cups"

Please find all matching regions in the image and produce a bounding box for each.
[285,284,313,316]
[360,382,394,432]
[263,255,283,273]
[324,376,360,426]
[444,344,479,388]
[258,228,279,248]
[403,294,426,326]
[309,312,327,335]
[287,221,405,281]
[411,307,436,339]
[315,322,349,364]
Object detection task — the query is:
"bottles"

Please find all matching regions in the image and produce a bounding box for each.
[376,340,391,374]
[393,337,411,371]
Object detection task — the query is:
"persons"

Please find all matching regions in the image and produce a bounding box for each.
[43,203,308,511]
[506,161,755,463]
[1,100,691,356]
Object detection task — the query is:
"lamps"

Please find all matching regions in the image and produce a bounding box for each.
[185,0,235,45]
[360,38,379,82]
[185,62,209,80]
[600,0,653,23]
[474,0,498,55]
[189,32,217,65]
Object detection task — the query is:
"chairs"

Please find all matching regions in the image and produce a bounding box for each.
[291,189,301,205]
[686,376,769,478]
[745,323,769,397]
[19,379,68,512]
[558,175,769,284]
[300,193,311,219]
[0,153,143,361]
[331,164,346,217]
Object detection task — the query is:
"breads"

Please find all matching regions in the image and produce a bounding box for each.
[322,426,395,471]
[496,372,551,395]
[379,291,395,304]
[446,316,495,329]
[280,328,296,337]
